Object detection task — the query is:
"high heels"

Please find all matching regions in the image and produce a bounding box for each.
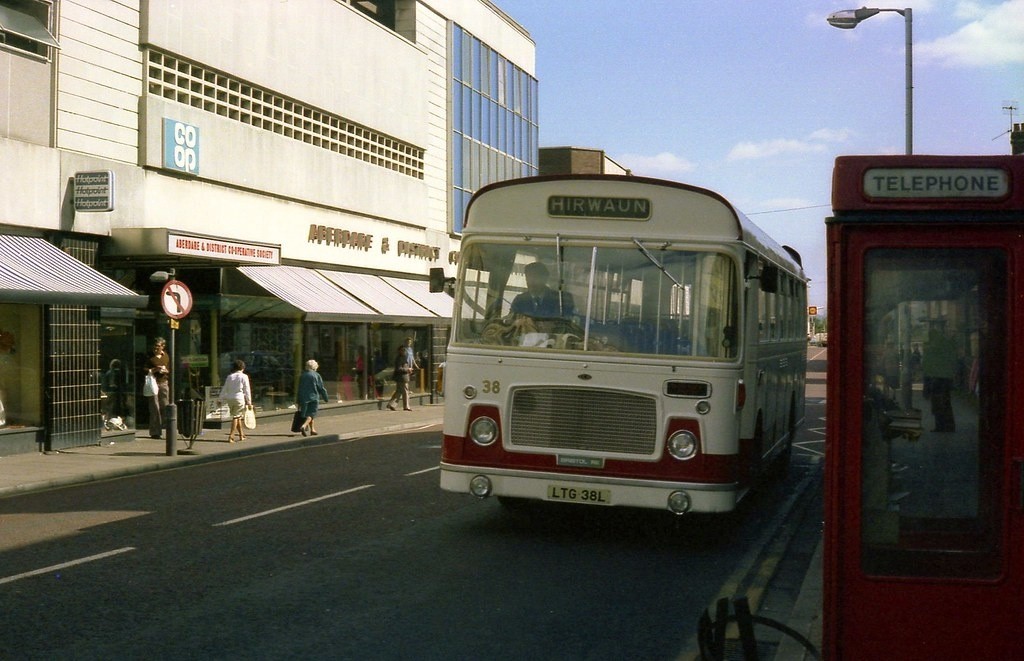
[229,436,235,443]
[240,435,246,441]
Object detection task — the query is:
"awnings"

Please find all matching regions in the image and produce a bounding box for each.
[0,235,148,306]
[237,266,524,326]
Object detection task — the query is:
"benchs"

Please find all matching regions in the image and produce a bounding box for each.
[377,392,434,410]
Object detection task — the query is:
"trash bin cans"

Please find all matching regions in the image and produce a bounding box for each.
[176,399,207,437]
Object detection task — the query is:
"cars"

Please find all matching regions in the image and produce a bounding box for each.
[219,350,290,392]
[807,333,828,348]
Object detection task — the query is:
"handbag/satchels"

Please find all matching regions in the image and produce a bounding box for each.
[143,369,159,397]
[244,404,256,429]
[291,405,309,432]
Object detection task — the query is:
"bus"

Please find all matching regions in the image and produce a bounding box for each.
[428,148,811,539]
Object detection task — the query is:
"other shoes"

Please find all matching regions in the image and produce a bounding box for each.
[404,408,411,411]
[387,404,394,410]
[151,434,160,438]
[311,431,318,435]
[302,426,307,437]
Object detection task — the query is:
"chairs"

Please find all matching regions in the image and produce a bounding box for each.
[579,314,679,353]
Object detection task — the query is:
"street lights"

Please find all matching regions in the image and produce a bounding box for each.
[825,5,918,157]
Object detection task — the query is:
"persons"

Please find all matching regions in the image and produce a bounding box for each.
[508,263,575,321]
[387,338,419,411]
[881,342,921,412]
[923,330,958,432]
[93,359,129,430]
[296,360,328,437]
[217,359,252,443]
[356,345,384,398]
[144,338,170,439]
